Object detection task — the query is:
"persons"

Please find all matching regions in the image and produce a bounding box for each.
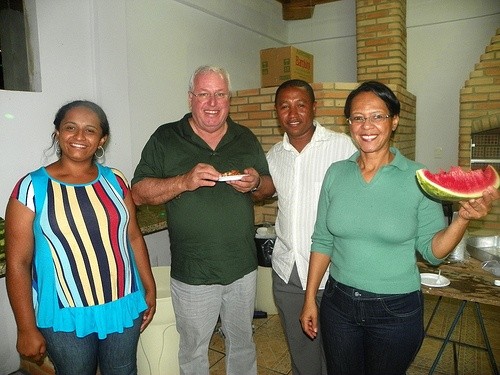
[131,65,275,375]
[300,81,500,375]
[265,79,357,375]
[5,101,156,375]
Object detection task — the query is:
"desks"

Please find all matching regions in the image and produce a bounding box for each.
[0,205,167,277]
[417,253,500,375]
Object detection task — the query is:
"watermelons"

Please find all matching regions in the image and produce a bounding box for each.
[416,164,500,201]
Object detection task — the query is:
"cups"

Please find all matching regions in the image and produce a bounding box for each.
[448,211,467,261]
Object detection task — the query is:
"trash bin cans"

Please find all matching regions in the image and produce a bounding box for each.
[254,224,279,315]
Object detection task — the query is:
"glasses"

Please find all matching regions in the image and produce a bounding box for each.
[346,112,395,126]
[189,91,231,104]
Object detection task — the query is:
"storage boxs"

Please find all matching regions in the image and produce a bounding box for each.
[260,46,314,87]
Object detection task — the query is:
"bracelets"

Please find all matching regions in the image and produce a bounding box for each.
[251,178,261,192]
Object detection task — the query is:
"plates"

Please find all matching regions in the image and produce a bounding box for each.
[418,272,451,287]
[217,174,251,181]
